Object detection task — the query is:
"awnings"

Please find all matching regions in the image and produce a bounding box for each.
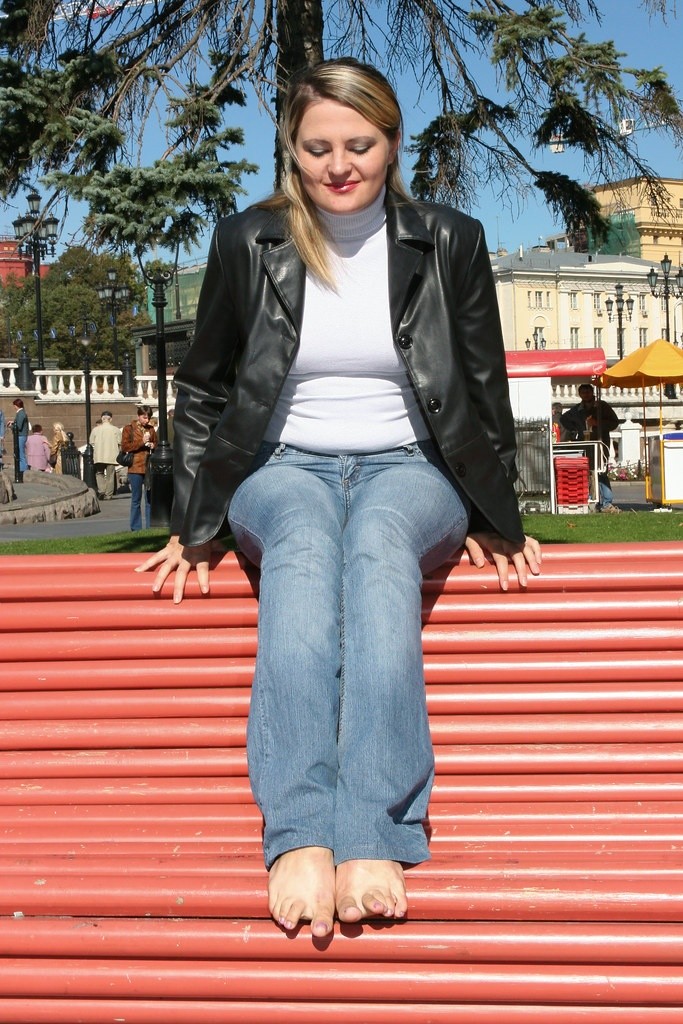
[502,347,608,473]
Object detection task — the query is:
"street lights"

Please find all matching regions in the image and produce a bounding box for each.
[135,218,180,529]
[647,252,682,399]
[96,267,131,369]
[525,333,547,350]
[73,315,100,492]
[605,282,636,362]
[13,191,59,393]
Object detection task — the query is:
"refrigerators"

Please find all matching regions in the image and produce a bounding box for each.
[648,433,683,500]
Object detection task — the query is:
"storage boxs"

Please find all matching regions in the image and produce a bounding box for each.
[554,457,590,516]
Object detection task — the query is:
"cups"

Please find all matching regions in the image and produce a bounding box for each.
[583,431,590,440]
[572,431,578,439]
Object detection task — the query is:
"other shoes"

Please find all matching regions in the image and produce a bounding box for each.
[601,504,621,512]
[99,493,105,499]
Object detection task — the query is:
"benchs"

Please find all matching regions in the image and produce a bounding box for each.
[0,543,683,1024]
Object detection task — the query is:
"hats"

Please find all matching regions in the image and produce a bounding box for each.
[102,411,112,417]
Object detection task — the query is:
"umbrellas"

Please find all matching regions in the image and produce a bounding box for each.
[592,336,683,504]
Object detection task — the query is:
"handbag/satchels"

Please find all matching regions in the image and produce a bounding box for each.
[48,452,58,466]
[45,464,55,474]
[116,425,134,465]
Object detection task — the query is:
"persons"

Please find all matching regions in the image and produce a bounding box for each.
[88,409,175,501]
[48,421,76,475]
[552,403,578,442]
[0,411,6,470]
[5,398,29,473]
[560,383,624,515]
[131,55,546,940]
[24,424,55,474]
[121,404,160,532]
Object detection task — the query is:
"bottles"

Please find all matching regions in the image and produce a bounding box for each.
[586,416,592,432]
